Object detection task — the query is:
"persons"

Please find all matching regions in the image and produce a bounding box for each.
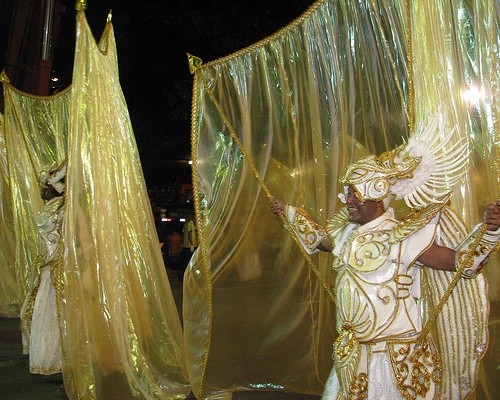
[153,210,200,271]
[19,160,69,384]
[268,155,499,400]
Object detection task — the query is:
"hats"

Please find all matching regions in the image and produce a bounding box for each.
[40,157,66,195]
[340,114,473,209]
